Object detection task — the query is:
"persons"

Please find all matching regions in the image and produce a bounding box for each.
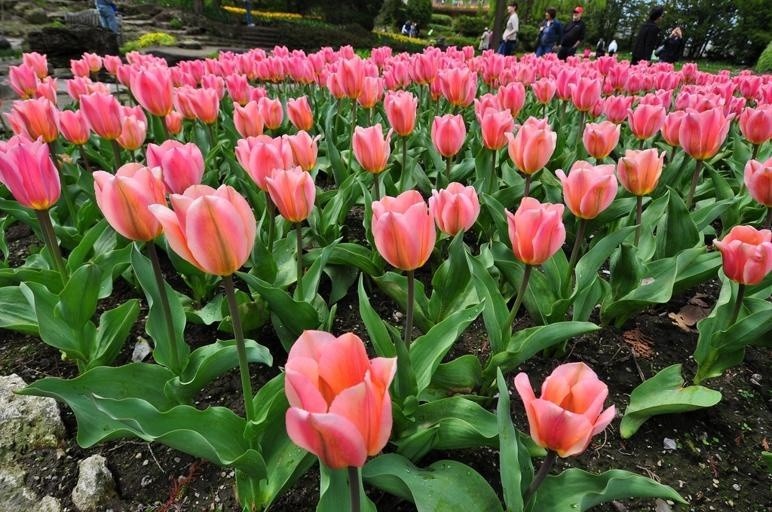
[583,46,591,61]
[94,0,122,49]
[487,29,494,49]
[607,38,618,57]
[497,2,519,57]
[554,6,585,64]
[244,0,256,28]
[594,37,606,60]
[655,27,683,65]
[535,7,563,57]
[629,4,664,67]
[478,27,489,56]
[382,12,423,40]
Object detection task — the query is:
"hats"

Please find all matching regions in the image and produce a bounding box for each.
[573,6,583,15]
